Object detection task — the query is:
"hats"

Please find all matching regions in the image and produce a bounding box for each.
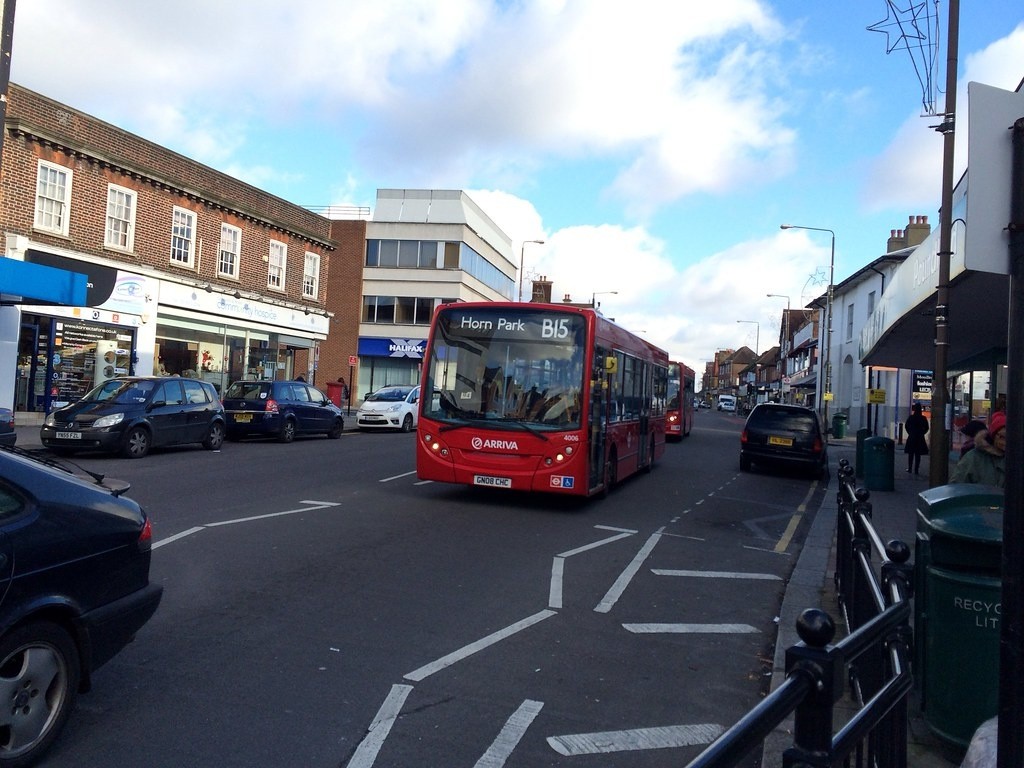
[961,420,988,438]
[988,412,1007,437]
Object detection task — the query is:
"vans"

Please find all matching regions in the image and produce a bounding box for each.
[719,395,734,404]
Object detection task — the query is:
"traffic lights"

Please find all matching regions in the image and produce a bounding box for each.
[746,383,752,396]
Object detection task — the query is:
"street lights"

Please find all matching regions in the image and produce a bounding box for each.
[781,224,834,421]
[768,294,790,402]
[592,291,618,310]
[737,320,759,406]
[519,240,545,301]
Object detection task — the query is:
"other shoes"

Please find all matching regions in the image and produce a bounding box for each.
[915,470,918,474]
[905,468,912,473]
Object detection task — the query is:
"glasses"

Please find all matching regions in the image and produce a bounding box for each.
[996,431,1007,441]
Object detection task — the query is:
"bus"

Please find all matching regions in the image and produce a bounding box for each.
[666,361,696,441]
[413,302,669,499]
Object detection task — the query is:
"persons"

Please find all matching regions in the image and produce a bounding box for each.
[948,412,1006,489]
[905,402,929,475]
[959,420,988,460]
[294,373,306,382]
[337,377,348,413]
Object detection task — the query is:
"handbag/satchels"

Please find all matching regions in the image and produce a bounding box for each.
[345,384,349,399]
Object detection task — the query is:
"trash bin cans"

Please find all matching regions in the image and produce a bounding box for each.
[862,437,895,491]
[832,413,848,439]
[365,393,374,400]
[856,428,869,478]
[910,484,1002,762]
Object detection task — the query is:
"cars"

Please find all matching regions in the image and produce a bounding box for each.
[722,402,735,412]
[737,403,754,416]
[0,444,163,768]
[740,401,826,479]
[717,402,724,411]
[0,408,17,451]
[40,376,229,455]
[222,380,345,443]
[694,399,711,412]
[355,384,441,433]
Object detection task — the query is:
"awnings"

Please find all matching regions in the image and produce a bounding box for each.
[790,372,816,389]
